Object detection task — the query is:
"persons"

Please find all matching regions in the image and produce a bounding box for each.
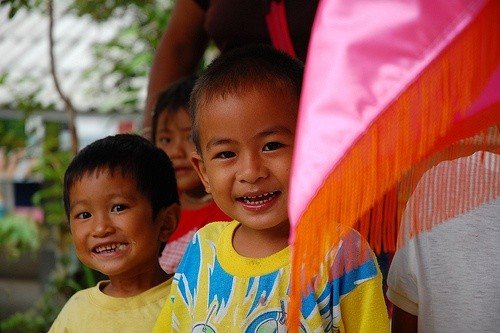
[386,127,500,333]
[150,76,235,274]
[47,134,182,333]
[154,42,390,333]
